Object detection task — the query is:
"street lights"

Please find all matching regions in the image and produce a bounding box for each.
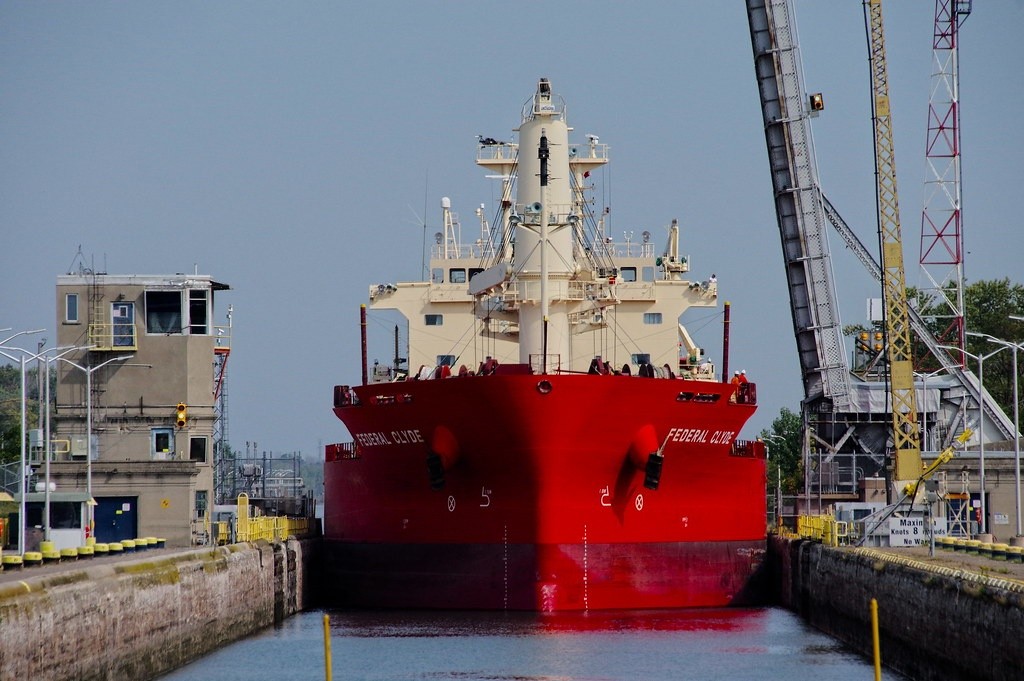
[965,315,1024,534]
[52,355,135,544]
[756,431,796,528]
[888,364,964,451]
[0,328,96,556]
[936,344,1012,533]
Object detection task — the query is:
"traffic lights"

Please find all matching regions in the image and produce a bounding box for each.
[176,402,187,427]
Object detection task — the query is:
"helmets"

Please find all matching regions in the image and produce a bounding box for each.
[741,370,745,374]
[734,371,739,374]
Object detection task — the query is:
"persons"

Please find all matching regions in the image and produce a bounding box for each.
[731,369,748,403]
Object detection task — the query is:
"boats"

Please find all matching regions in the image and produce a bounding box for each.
[324,79,766,615]
